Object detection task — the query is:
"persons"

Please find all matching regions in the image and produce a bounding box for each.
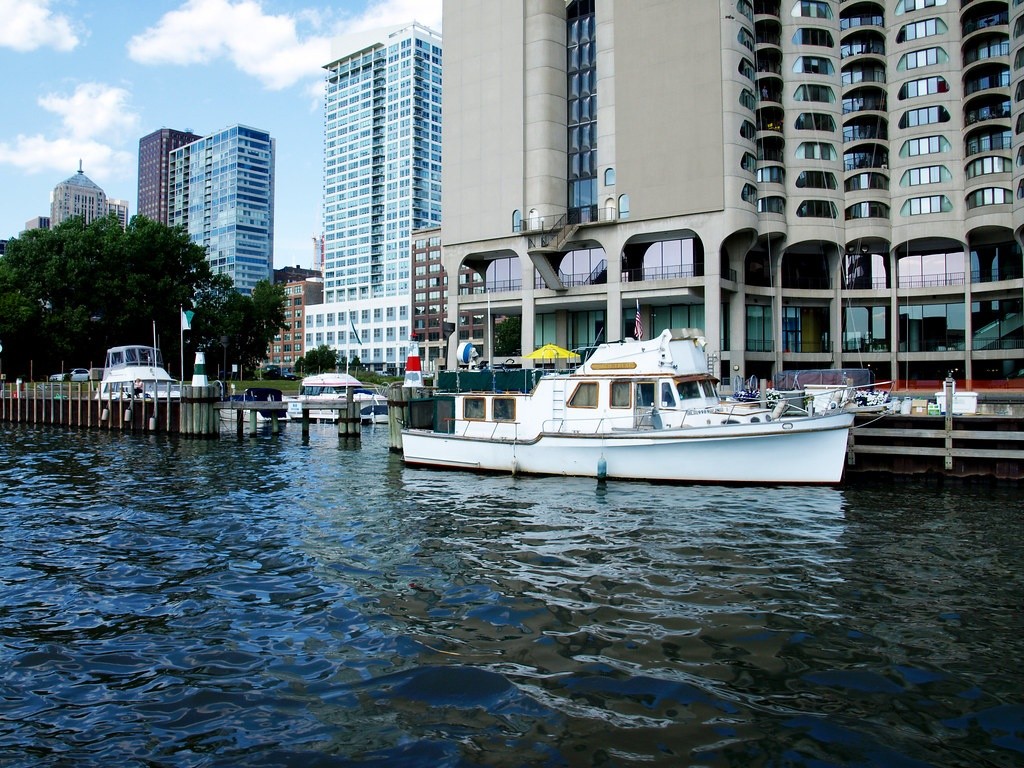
[134,378,144,398]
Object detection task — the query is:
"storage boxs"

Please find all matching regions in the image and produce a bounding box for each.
[928,404,941,416]
[935,392,979,414]
[911,400,929,415]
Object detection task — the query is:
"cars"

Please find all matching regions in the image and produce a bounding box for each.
[49,370,70,382]
[262,368,297,381]
[66,367,90,382]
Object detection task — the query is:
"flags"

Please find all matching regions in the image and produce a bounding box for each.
[182,310,194,330]
[634,303,643,338]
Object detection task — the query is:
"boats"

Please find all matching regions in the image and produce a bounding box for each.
[94,344,389,422]
[400,326,898,486]
